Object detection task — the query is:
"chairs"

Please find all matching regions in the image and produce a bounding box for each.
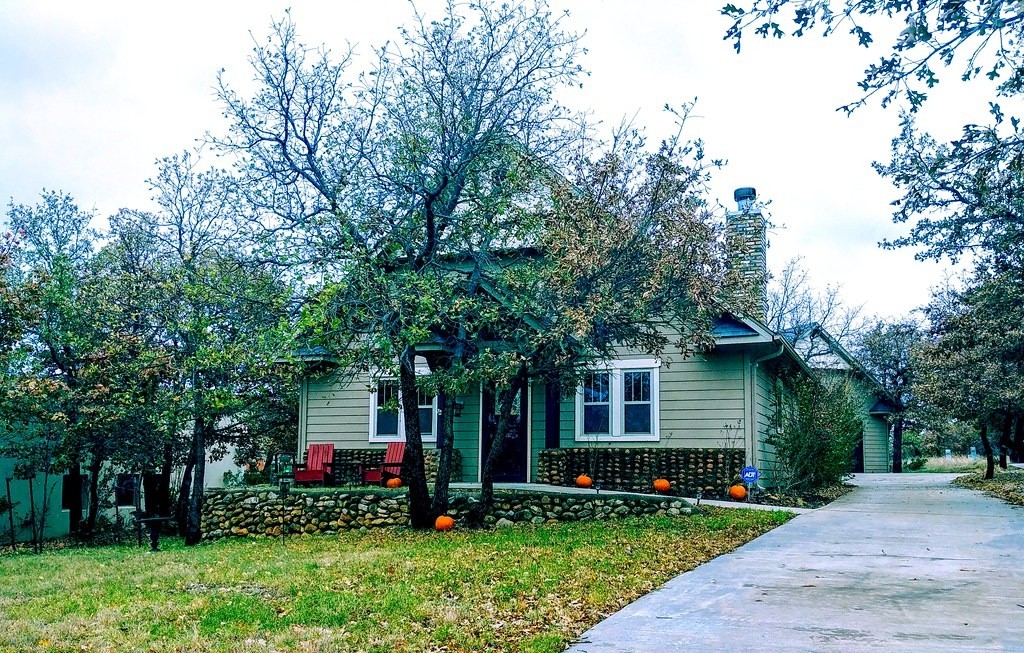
[293,444,336,487]
[361,444,408,488]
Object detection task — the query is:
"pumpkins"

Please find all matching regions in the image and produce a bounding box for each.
[434,515,454,531]
[576,475,592,488]
[730,485,746,498]
[386,477,402,488]
[653,478,670,491]
